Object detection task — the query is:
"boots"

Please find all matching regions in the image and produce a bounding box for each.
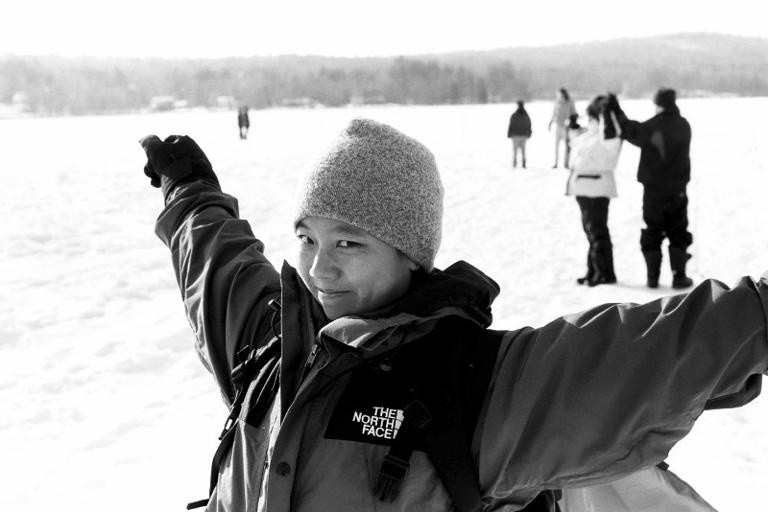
[642,250,692,288]
[577,247,616,286]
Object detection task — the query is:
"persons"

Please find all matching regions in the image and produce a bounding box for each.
[564,110,588,199]
[546,85,579,170]
[134,114,766,511]
[237,104,250,141]
[606,85,695,290]
[564,93,624,289]
[506,99,533,169]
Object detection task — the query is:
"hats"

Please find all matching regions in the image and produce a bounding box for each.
[654,88,675,108]
[587,96,604,122]
[296,120,443,272]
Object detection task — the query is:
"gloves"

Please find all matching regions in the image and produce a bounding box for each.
[570,113,580,128]
[599,93,621,119]
[139,135,219,204]
